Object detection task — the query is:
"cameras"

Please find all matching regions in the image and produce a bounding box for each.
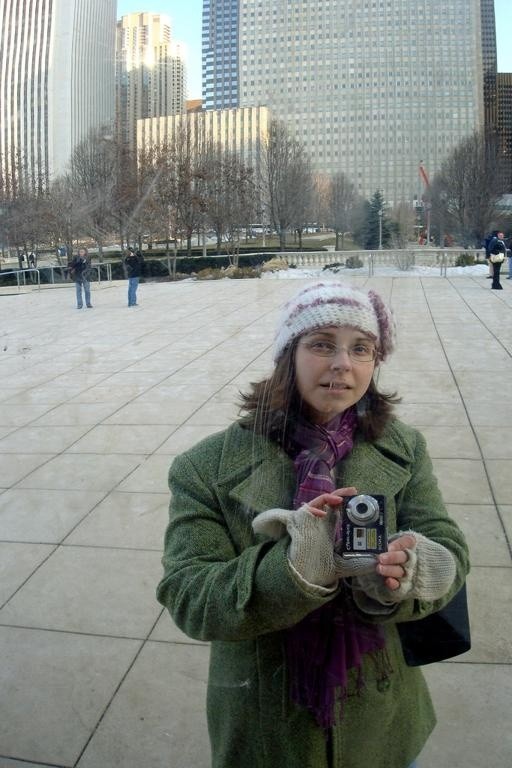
[341,494,388,554]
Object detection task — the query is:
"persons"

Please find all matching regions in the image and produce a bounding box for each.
[19,253,25,262]
[67,247,94,309]
[419,231,424,246]
[444,233,454,247]
[505,235,512,279]
[484,227,495,278]
[153,277,471,768]
[28,253,35,269]
[124,246,144,306]
[487,231,507,290]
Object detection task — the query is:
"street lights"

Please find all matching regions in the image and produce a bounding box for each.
[378,210,382,250]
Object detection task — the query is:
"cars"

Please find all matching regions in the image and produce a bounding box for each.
[232,224,280,237]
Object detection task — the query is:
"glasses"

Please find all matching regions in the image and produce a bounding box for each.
[294,339,382,362]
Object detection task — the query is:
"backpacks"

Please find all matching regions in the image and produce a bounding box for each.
[394,579,472,667]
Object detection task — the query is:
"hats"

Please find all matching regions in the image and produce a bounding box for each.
[274,281,394,365]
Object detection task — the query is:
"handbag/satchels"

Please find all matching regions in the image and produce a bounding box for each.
[490,252,504,263]
